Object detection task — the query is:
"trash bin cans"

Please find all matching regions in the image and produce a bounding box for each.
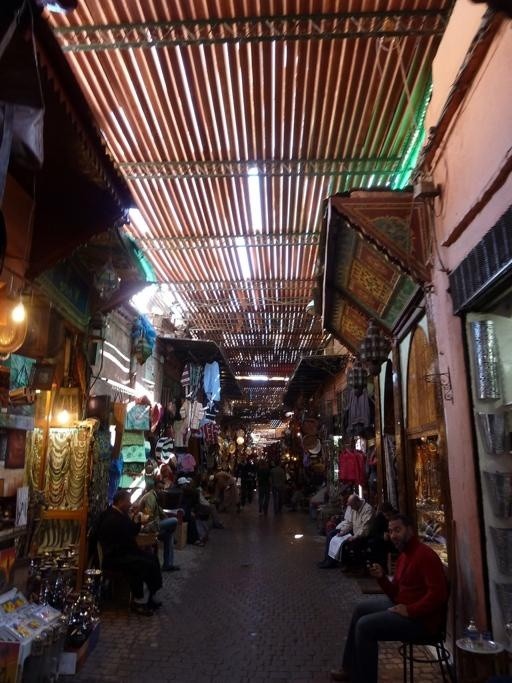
[456,638,505,683]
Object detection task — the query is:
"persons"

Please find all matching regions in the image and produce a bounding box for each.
[138,479,181,573]
[95,489,163,610]
[328,512,450,682]
[156,448,283,548]
[314,493,374,569]
[374,502,401,575]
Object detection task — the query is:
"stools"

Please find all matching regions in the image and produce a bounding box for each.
[104,562,145,616]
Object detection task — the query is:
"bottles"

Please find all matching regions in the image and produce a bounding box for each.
[469,318,502,402]
[23,614,67,682]
[463,618,494,648]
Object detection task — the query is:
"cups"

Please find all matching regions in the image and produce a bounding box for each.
[477,402,511,636]
[87,394,112,419]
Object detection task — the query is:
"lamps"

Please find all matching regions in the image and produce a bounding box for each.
[93,230,121,303]
[347,352,369,398]
[356,317,391,376]
[412,179,442,201]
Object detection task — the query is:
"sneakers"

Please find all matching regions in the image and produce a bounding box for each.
[131,598,162,616]
[330,665,349,681]
[317,559,339,569]
[161,562,182,572]
[193,519,225,547]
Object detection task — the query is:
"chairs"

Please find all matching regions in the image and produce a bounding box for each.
[394,591,451,682]
